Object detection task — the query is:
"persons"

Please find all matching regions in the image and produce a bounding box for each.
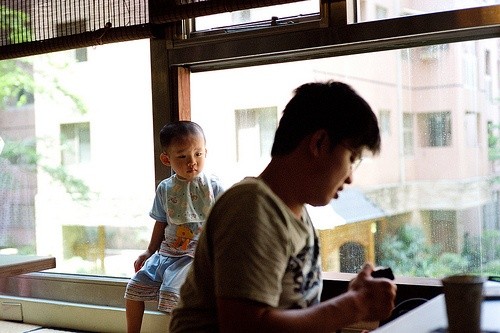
[167,79,400,333]
[123,120,226,333]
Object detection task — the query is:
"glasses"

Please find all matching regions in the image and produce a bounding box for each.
[336,139,363,170]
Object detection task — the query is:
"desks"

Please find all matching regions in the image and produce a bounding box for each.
[367,274,500,332]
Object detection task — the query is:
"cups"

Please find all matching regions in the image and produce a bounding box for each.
[441,274,483,333]
